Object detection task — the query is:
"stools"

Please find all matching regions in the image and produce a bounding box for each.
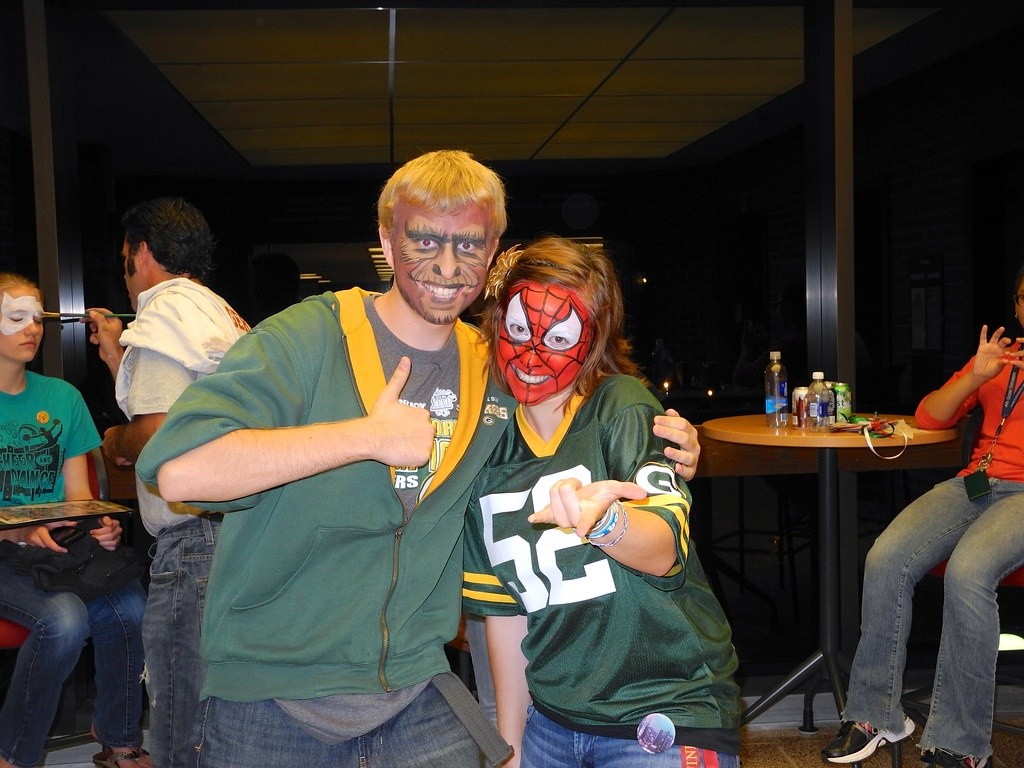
[891,553,1024,768]
[0,439,110,650]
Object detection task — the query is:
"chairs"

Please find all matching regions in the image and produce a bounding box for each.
[92,445,141,507]
[444,615,476,696]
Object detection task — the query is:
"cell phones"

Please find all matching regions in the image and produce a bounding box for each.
[49,526,85,547]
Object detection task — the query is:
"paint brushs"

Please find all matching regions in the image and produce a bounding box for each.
[35,311,136,318]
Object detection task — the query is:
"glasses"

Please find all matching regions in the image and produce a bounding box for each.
[1015,295,1024,306]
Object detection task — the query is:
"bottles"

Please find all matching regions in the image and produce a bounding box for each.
[807,372,830,432]
[765,352,788,427]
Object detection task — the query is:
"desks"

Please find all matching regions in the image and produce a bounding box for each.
[701,413,962,742]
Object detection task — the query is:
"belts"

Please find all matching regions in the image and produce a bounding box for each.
[432,671,515,768]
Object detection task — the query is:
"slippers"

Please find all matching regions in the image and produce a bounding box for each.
[92,749,149,768]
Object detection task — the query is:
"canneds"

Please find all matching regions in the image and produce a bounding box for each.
[792,381,852,429]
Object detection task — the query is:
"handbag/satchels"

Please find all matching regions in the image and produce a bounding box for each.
[829,418,908,459]
[0,521,143,601]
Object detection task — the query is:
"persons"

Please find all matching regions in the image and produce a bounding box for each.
[0,146,747,768]
[824,262,1023,768]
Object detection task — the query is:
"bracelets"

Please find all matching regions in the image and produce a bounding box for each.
[572,499,629,551]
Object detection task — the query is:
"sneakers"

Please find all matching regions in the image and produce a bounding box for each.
[821,713,916,764]
[926,746,989,768]
